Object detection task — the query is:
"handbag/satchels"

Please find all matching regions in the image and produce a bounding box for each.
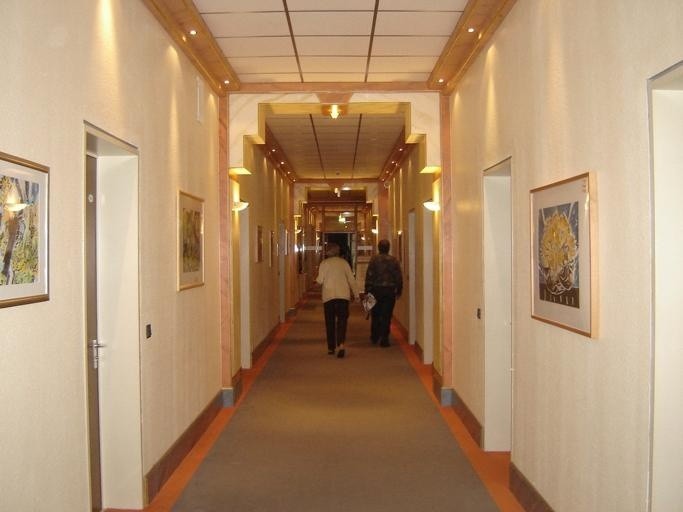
[362,293,377,312]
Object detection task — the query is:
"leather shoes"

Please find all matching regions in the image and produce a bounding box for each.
[336,350,344,357]
[328,349,334,354]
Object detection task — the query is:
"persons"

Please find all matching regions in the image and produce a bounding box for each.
[363,239,402,348]
[313,241,361,358]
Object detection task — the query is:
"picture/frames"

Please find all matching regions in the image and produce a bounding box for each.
[0,149,50,310]
[527,170,602,340]
[255,224,263,263]
[174,188,205,293]
